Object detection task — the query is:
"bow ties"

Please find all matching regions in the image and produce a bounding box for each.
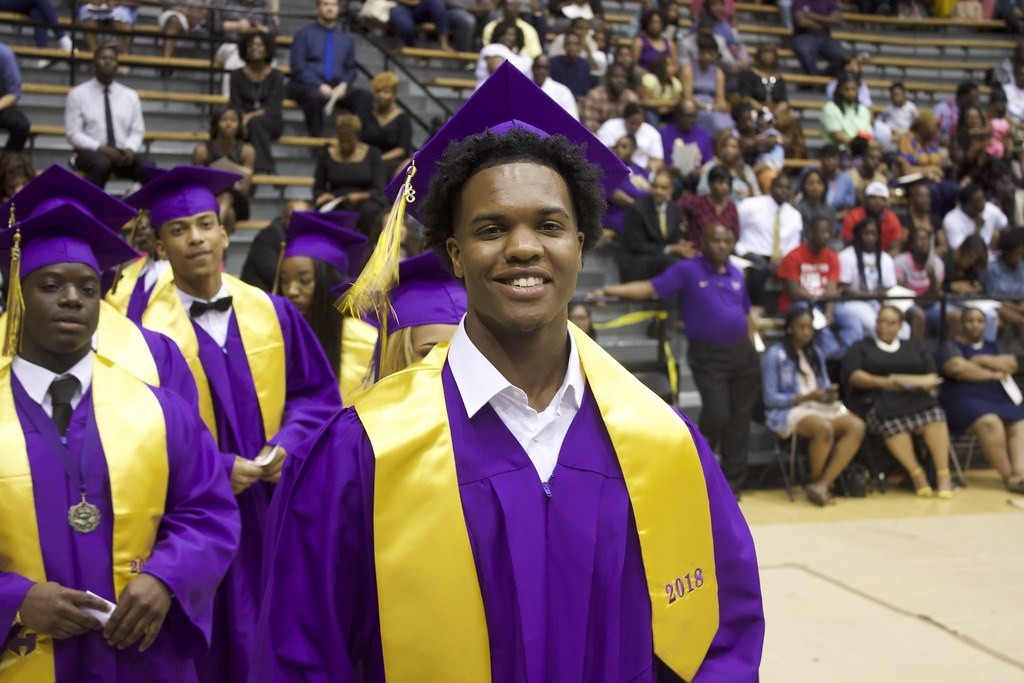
[190,295,233,318]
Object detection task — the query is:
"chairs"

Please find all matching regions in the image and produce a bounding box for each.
[755,346,976,501]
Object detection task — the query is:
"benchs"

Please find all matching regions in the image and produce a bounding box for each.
[0,0,1024,329]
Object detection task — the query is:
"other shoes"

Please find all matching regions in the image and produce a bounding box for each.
[1007,476,1024,492]
[939,471,952,499]
[806,482,828,505]
[911,468,932,499]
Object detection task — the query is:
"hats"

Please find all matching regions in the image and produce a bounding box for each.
[326,247,471,384]
[864,181,890,200]
[1,163,139,235]
[0,202,139,356]
[283,211,369,277]
[121,165,246,230]
[336,60,631,317]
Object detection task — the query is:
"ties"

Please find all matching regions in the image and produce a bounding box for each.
[103,88,117,148]
[772,209,780,265]
[325,28,336,85]
[659,208,667,238]
[47,376,79,437]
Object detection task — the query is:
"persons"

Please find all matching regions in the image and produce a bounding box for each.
[781,217,864,357]
[680,167,741,254]
[330,244,469,382]
[893,229,962,344]
[839,306,952,502]
[616,169,695,339]
[246,58,766,683]
[0,0,410,213]
[354,0,1024,248]
[939,227,1024,353]
[110,163,341,683]
[272,209,382,405]
[839,218,911,341]
[585,224,756,508]
[569,303,595,339]
[734,173,803,313]
[759,309,867,509]
[0,164,242,683]
[938,309,1024,494]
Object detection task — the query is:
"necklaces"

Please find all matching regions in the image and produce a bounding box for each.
[10,381,100,533]
